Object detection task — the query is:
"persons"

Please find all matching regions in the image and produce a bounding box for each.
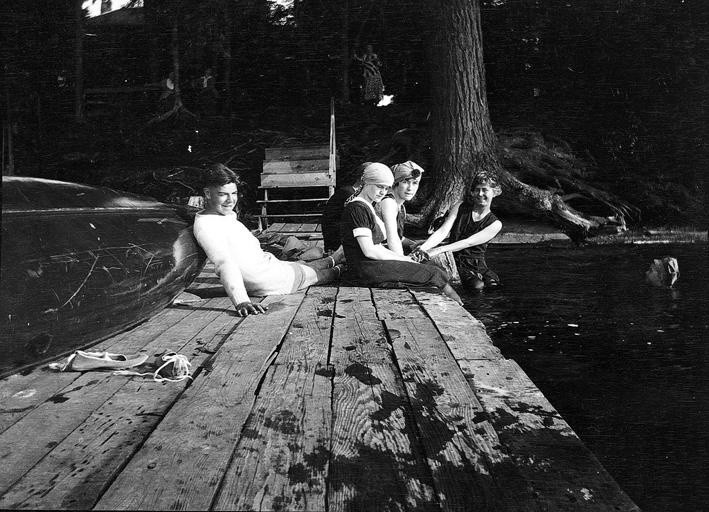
[321,162,373,257]
[340,160,465,306]
[374,160,462,286]
[411,169,503,289]
[193,163,347,317]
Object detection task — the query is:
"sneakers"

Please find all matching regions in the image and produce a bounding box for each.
[155,348,188,379]
[70,350,149,372]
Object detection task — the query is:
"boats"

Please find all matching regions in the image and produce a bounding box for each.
[1,173,208,377]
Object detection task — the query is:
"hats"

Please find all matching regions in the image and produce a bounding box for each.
[389,160,425,190]
[653,256,680,286]
